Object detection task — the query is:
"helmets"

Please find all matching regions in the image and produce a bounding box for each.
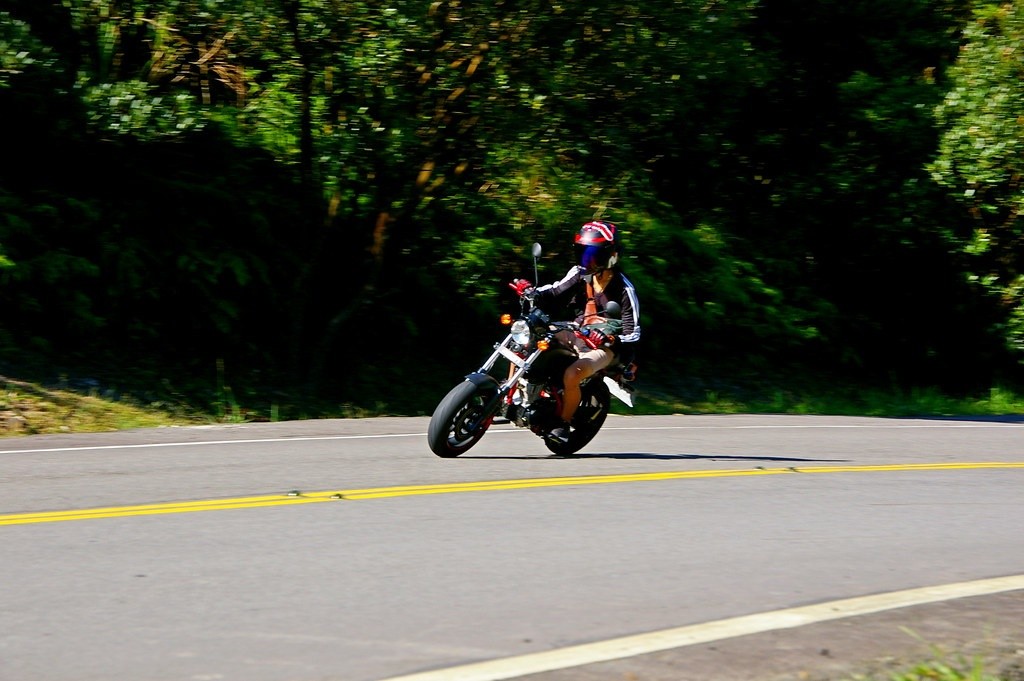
[573,220,617,275]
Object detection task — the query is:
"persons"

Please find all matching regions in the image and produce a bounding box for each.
[514,221,642,446]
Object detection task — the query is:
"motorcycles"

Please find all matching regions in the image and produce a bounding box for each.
[428,240,638,458]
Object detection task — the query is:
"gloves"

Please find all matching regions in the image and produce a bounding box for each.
[583,328,608,349]
[515,278,532,296]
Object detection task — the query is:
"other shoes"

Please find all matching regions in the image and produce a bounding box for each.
[550,421,570,442]
